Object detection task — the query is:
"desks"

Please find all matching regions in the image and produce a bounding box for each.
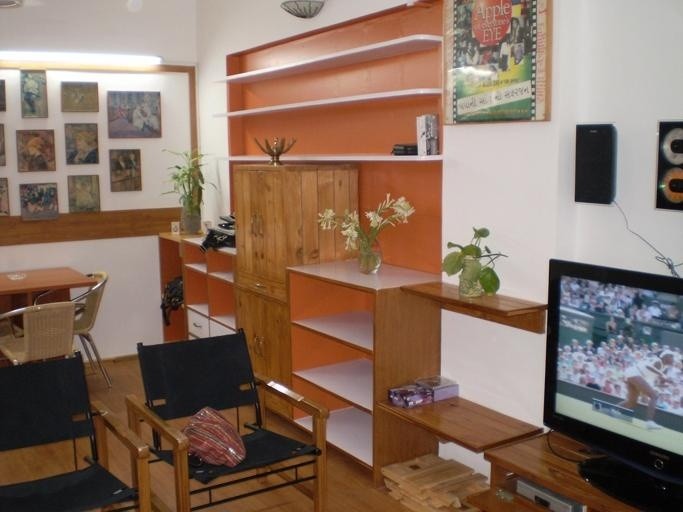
[0,267,97,311]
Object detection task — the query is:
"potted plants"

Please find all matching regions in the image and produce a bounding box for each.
[440,224,511,299]
[163,149,220,239]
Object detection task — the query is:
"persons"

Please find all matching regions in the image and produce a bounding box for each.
[557,335,682,429]
[561,277,678,333]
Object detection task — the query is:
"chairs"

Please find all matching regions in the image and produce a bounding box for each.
[0,353,152,512]
[121,326,335,512]
[0,302,76,365]
[33,271,114,388]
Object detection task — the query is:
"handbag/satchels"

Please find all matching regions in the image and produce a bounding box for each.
[181,406,246,468]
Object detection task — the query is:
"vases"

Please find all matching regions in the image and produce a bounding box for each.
[354,243,382,276]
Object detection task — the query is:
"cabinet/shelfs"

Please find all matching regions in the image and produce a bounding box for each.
[484,431,651,512]
[229,162,360,425]
[375,285,565,512]
[290,257,443,474]
[224,0,444,277]
[157,229,235,342]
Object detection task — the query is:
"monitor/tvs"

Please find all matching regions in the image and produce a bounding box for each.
[542,258,683,512]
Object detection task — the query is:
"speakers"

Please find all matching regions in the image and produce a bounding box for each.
[575,125,618,205]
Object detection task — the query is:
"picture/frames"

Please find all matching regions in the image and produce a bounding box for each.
[0,123,7,168]
[59,80,100,114]
[0,177,11,217]
[0,79,7,114]
[64,122,99,167]
[19,69,49,120]
[106,90,162,140]
[15,128,57,173]
[108,148,142,192]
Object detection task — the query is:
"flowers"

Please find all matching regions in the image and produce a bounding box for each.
[315,190,415,249]
